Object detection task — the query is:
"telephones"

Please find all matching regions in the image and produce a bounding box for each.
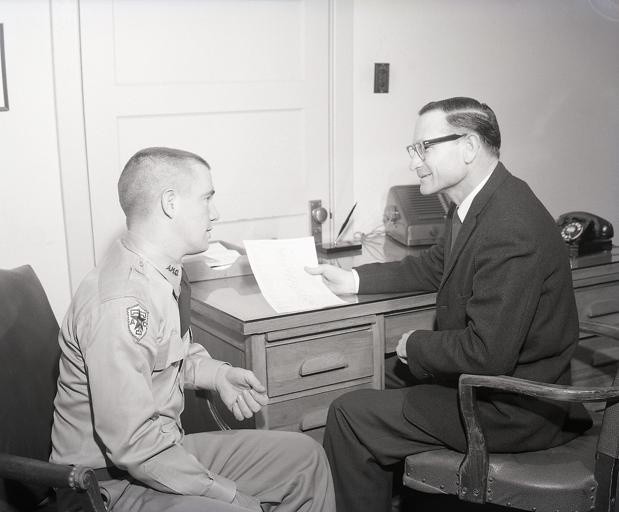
[556,211,614,257]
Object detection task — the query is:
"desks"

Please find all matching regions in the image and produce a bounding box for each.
[180,233,619,445]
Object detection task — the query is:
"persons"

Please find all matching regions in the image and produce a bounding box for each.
[49,148,337,512]
[304,97,593,512]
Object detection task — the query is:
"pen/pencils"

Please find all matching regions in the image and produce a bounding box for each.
[333,200,359,243]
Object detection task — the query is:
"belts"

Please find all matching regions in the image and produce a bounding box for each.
[94,465,128,481]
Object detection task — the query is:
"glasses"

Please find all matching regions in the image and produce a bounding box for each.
[405,133,466,162]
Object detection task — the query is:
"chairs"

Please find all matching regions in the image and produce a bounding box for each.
[402,320,619,512]
[1,262,230,511]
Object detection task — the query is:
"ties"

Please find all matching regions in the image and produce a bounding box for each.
[450,207,463,252]
